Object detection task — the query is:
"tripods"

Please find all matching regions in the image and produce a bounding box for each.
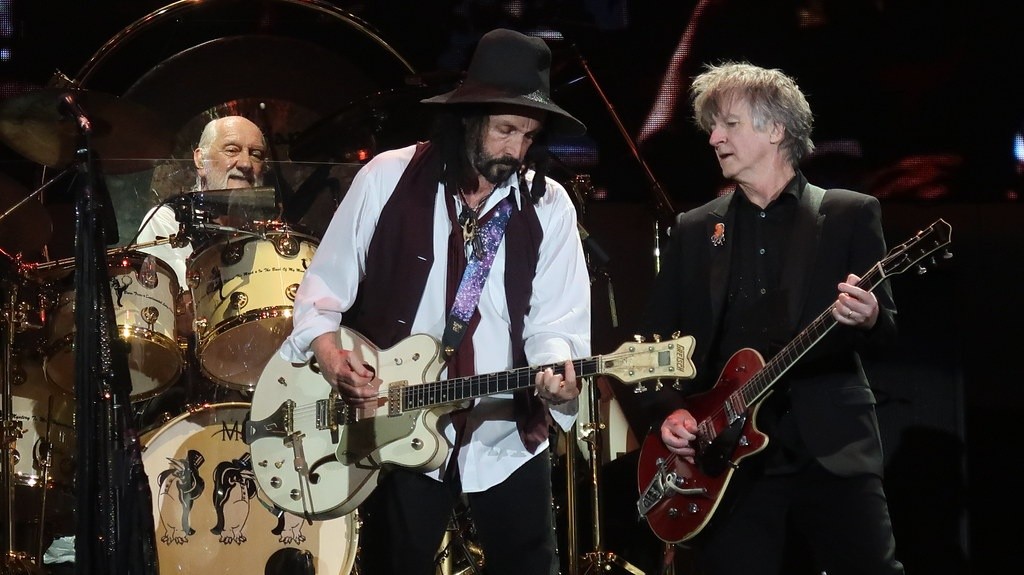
[575,378,648,575]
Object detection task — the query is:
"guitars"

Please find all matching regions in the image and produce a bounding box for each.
[634,216,956,548]
[245,322,695,523]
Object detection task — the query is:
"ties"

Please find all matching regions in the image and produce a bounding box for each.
[445,183,496,438]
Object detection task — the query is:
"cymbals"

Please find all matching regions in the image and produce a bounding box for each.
[0,84,86,169]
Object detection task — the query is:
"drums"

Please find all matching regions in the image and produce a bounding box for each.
[182,225,314,392]
[136,402,364,575]
[0,388,79,494]
[38,246,183,404]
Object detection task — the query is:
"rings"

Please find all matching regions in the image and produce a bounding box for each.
[848,310,854,319]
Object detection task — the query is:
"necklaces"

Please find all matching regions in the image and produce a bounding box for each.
[457,183,498,259]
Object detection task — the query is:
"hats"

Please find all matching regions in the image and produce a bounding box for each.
[420,28,588,140]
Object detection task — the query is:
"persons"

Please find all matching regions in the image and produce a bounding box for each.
[135,115,270,429]
[632,64,905,575]
[280,28,591,575]
